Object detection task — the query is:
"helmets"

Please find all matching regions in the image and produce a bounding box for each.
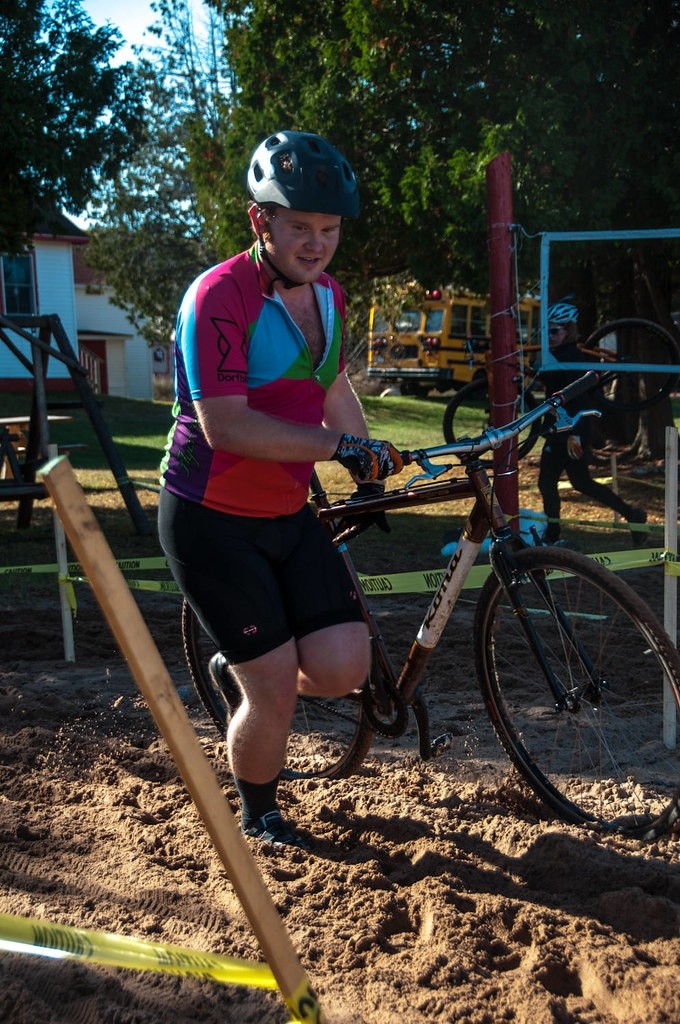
[244,130,358,217]
[548,303,578,323]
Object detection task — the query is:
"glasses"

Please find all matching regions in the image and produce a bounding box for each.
[546,326,564,335]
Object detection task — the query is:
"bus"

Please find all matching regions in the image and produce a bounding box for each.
[367,288,540,401]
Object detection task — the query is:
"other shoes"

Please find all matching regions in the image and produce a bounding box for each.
[209,651,243,724]
[240,809,302,846]
[627,508,647,547]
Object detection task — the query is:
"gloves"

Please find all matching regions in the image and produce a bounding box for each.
[330,433,403,480]
[329,482,391,550]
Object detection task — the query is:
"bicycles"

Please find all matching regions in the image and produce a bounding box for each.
[182,372,680,842]
[442,318,680,469]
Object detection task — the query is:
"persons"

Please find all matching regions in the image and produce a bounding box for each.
[537,304,647,547]
[156,133,400,839]
